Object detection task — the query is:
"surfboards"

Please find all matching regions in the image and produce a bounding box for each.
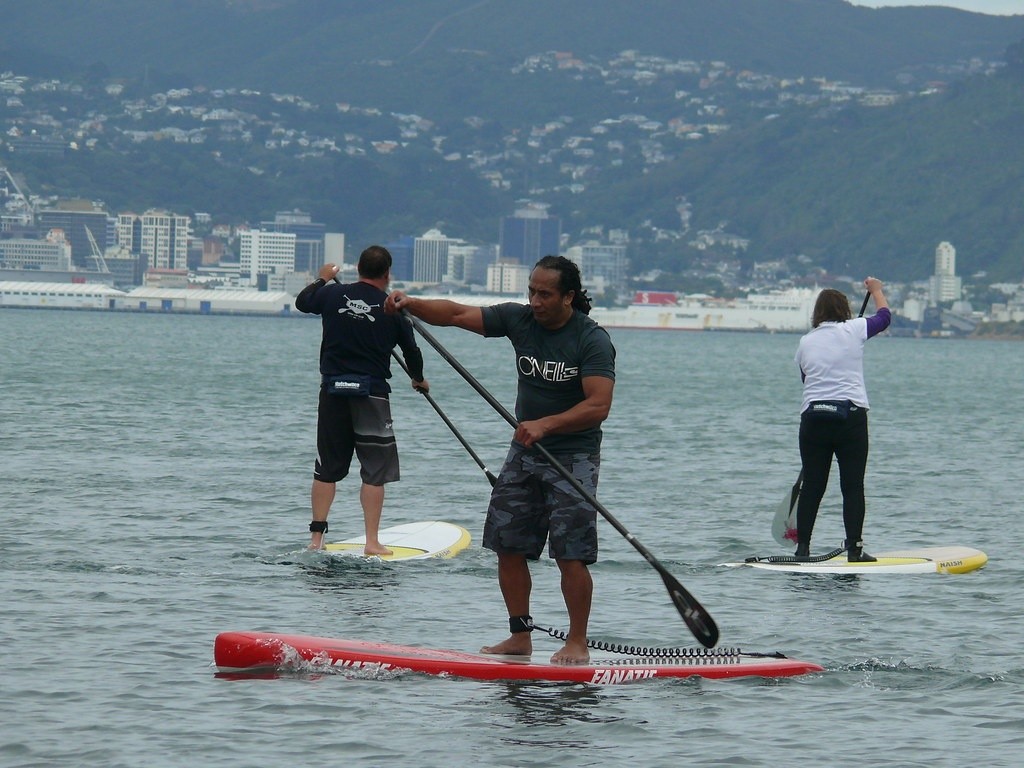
[715,543,990,577]
[275,518,473,576]
[207,626,825,689]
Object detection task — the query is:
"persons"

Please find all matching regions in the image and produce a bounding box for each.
[384,254,616,665]
[794,275,891,562]
[295,245,429,556]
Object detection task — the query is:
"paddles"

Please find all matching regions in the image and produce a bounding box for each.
[770,287,872,550]
[396,305,723,648]
[330,263,499,489]
